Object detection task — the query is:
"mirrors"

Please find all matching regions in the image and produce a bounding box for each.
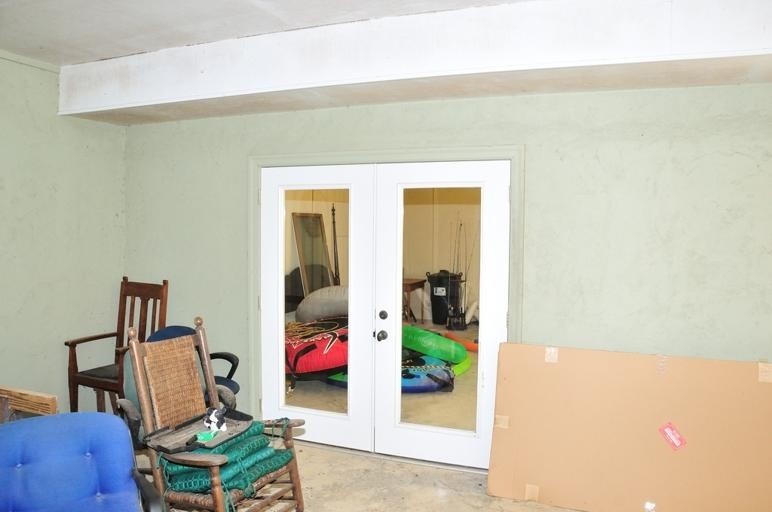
[291,211,334,298]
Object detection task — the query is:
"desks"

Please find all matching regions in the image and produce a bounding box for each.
[404,278,427,324]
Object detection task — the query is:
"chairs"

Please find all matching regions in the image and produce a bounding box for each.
[0,411,164,512]
[115,317,306,512]
[64,276,169,422]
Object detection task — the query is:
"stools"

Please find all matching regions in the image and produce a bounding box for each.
[295,284,349,323]
